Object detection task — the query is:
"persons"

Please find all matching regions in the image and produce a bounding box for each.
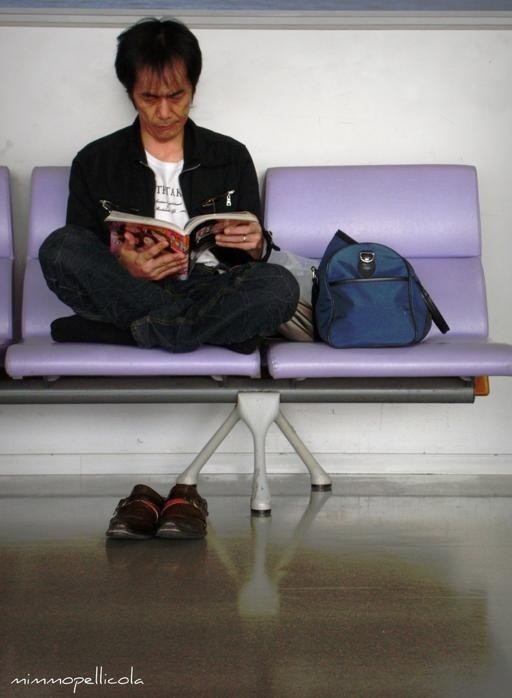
[38,21,301,350]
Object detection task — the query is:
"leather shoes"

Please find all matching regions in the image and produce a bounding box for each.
[106,484,165,540]
[154,483,209,540]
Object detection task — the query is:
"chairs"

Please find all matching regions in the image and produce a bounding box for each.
[2,163,512,513]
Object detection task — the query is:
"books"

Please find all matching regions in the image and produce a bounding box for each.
[104,208,260,282]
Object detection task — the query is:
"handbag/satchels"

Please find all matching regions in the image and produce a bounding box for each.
[312,229,450,348]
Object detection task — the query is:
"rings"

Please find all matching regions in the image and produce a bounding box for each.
[242,235,248,243]
